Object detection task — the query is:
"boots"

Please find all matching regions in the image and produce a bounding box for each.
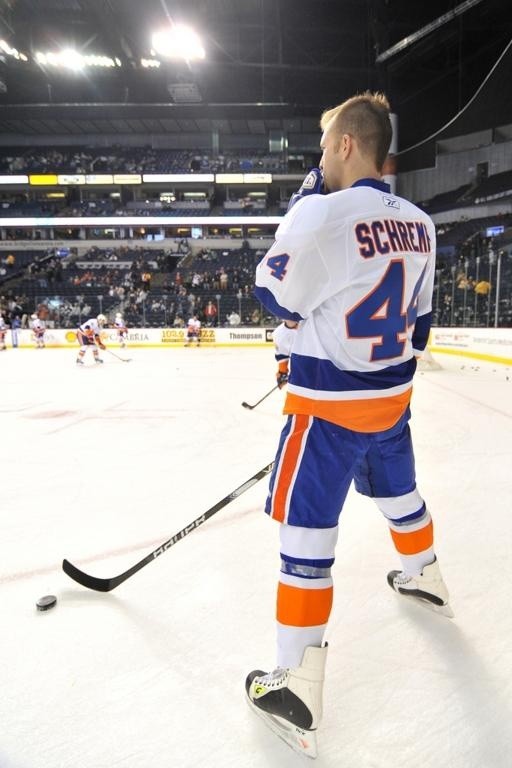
[387,553,450,606]
[244,641,329,734]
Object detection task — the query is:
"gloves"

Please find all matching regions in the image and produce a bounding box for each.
[286,168,326,214]
[276,370,289,391]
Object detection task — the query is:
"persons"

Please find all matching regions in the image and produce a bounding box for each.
[2,318,10,350]
[115,313,128,348]
[240,88,455,762]
[273,319,298,389]
[30,314,45,348]
[76,314,107,364]
[184,315,201,347]
[433,212,511,327]
[2,143,276,328]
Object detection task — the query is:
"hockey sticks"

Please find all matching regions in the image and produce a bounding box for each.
[241,380,287,410]
[62,461,275,592]
[105,348,132,363]
